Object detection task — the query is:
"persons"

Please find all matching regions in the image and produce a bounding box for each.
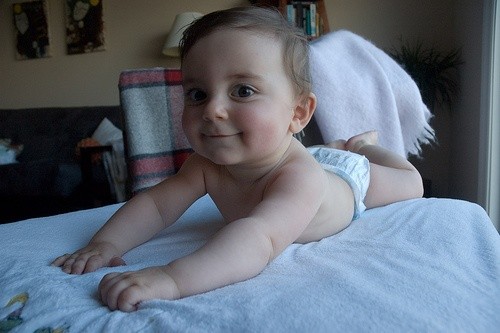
[50,5,424,312]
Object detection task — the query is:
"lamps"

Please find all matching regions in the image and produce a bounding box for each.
[162,11,205,57]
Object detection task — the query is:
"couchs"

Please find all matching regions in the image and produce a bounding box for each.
[0,105,127,193]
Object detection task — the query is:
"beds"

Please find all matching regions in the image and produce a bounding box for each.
[0,193,500,333]
